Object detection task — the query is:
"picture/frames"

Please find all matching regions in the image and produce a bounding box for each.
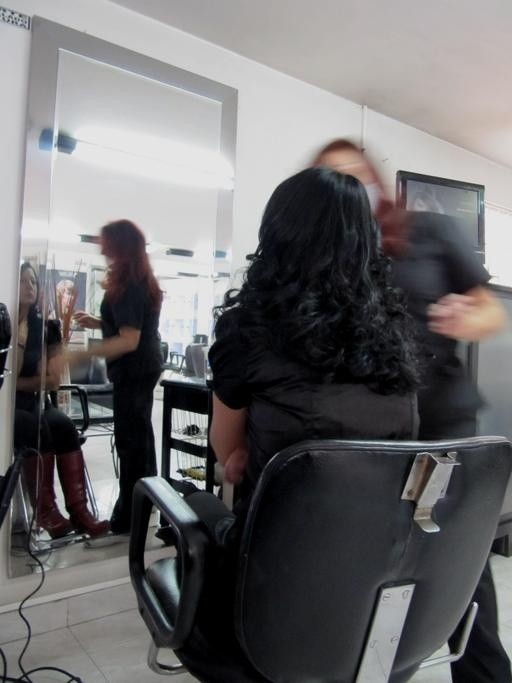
[395,168,488,254]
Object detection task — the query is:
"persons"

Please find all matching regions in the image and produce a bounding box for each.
[48,279,83,331]
[312,136,511,682]
[174,162,426,630]
[71,218,166,536]
[15,260,113,539]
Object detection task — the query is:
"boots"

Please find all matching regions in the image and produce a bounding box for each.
[19,447,74,539]
[54,448,112,538]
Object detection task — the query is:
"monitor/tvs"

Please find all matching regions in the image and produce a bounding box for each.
[395,170,486,267]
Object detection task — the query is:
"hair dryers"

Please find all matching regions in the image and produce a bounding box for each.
[0,302,12,390]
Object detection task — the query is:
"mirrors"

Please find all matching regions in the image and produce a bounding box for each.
[6,12,241,623]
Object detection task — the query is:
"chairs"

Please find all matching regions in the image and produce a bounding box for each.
[127,434,512,682]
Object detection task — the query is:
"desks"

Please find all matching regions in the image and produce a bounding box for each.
[159,375,214,528]
